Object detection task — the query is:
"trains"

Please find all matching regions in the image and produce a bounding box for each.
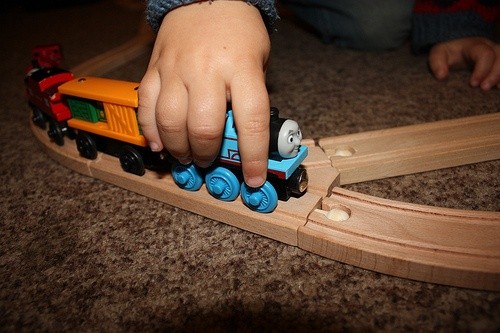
[24,43,309,213]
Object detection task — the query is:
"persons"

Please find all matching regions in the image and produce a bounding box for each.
[135,0,500,187]
